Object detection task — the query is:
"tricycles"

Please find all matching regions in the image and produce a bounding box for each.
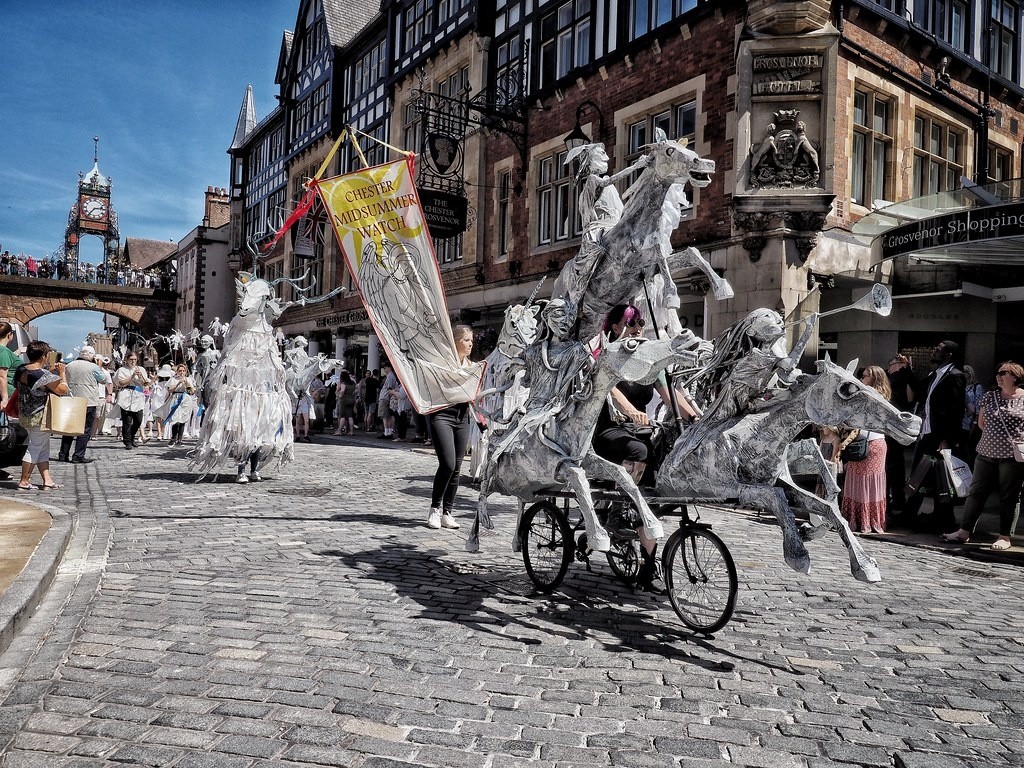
[519,418,739,635]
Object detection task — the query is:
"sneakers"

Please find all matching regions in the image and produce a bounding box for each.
[428,507,441,529]
[441,511,460,528]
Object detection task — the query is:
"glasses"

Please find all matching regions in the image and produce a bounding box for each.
[623,318,645,327]
[861,374,869,380]
[995,371,1010,376]
[933,346,947,355]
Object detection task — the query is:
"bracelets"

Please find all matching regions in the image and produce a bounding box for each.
[688,415,697,424]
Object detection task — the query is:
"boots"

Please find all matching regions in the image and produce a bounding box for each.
[885,496,956,534]
[605,487,639,540]
[636,542,670,596]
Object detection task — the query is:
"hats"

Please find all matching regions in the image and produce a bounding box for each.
[156,364,175,377]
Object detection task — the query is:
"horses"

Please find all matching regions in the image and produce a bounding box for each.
[463,126,924,586]
[185,270,345,484]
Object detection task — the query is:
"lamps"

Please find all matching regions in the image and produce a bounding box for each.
[563,99,610,152]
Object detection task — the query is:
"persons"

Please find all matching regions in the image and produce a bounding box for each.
[961,365,984,462]
[942,363,1024,550]
[842,366,892,534]
[0,322,204,480]
[284,336,309,373]
[593,305,698,592]
[192,335,220,384]
[487,298,595,430]
[889,340,966,531]
[888,358,916,511]
[426,324,477,528]
[57,345,106,464]
[0,251,172,290]
[700,308,819,421]
[561,143,650,300]
[814,427,841,500]
[223,278,271,484]
[13,340,67,491]
[295,362,432,446]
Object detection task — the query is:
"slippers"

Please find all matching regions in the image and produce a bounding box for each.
[990,540,1011,549]
[940,532,968,543]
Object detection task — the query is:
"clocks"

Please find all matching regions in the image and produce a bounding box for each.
[81,196,107,220]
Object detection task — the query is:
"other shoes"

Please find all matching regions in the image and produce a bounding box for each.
[16,482,39,491]
[236,472,249,483]
[72,456,93,463]
[59,457,69,462]
[250,470,261,482]
[90,430,192,450]
[890,497,906,510]
[43,482,64,490]
[295,423,434,447]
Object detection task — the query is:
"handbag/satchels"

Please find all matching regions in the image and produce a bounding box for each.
[5,388,19,417]
[840,438,868,461]
[1012,437,1024,462]
[0,411,16,453]
[40,384,88,437]
[904,448,973,507]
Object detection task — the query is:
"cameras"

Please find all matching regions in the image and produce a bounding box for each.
[55,364,59,367]
[92,358,98,363]
